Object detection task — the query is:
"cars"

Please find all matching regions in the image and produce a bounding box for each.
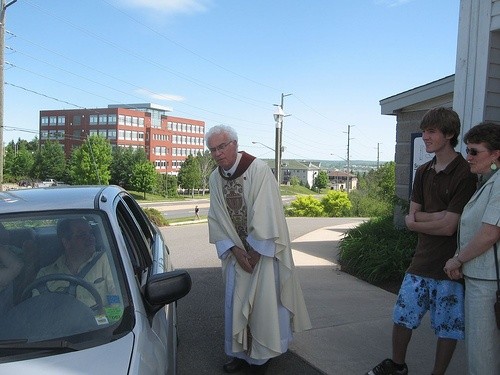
[0,185,193,375]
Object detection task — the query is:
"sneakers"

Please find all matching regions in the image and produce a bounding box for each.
[367,358,408,375]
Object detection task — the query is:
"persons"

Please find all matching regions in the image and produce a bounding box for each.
[32,217,121,315]
[0,222,35,314]
[364,107,478,375]
[193,205,201,221]
[443,120,500,375]
[205,124,313,375]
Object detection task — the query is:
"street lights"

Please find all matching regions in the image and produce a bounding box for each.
[272,105,284,187]
[330,153,349,194]
[252,141,282,188]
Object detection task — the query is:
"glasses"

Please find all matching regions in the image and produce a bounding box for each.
[466,147,495,156]
[209,140,234,152]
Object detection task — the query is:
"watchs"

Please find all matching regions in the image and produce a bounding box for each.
[455,252,463,264]
